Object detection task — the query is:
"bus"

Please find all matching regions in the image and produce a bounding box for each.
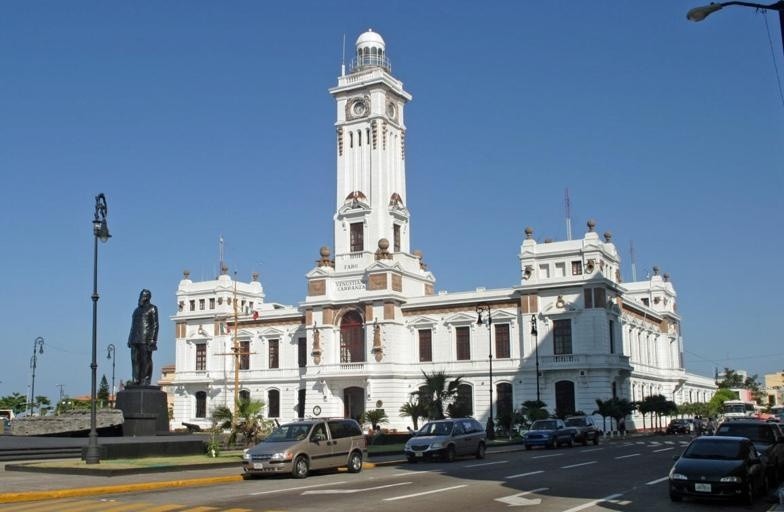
[721,401,783,421]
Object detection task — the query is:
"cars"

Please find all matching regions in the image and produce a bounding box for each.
[668,415,784,507]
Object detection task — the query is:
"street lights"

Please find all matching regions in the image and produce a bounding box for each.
[26,337,45,416]
[86,192,112,463]
[107,344,115,405]
[531,313,540,416]
[476,304,495,441]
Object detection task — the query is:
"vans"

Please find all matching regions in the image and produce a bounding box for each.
[403,418,486,462]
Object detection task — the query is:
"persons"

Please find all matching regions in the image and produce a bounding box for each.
[692,414,719,436]
[375,426,381,432]
[619,416,626,440]
[407,426,414,434]
[126,289,159,385]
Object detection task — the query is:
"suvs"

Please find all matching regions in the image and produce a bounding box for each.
[242,417,366,479]
[525,417,605,449]
